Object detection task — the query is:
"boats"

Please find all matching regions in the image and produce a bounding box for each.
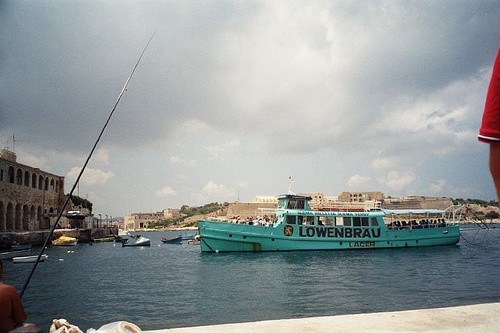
[13,253,49,263]
[196,176,461,253]
[94,237,115,242]
[122,234,151,247]
[160,235,183,245]
[53,236,77,246]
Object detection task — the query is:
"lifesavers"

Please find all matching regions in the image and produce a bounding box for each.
[273,215,278,222]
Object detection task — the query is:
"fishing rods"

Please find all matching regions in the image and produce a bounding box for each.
[19,27,159,299]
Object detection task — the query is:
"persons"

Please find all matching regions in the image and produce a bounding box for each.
[478,48,500,199]
[228,214,278,227]
[0,259,28,333]
[388,218,446,229]
